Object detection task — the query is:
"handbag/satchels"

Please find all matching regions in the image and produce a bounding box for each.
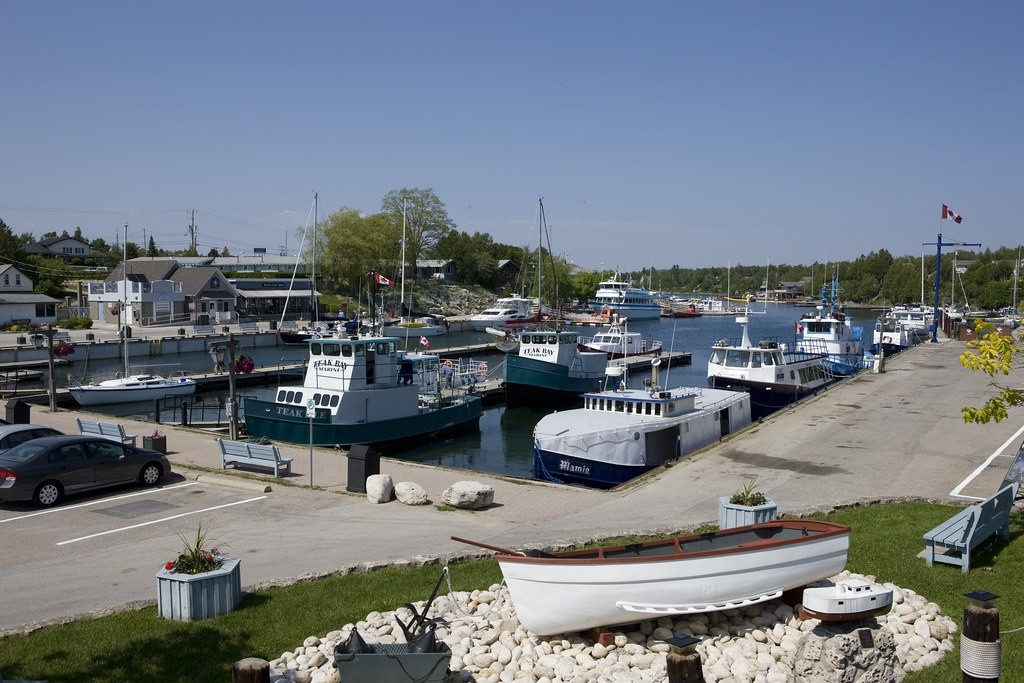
[218,361,225,366]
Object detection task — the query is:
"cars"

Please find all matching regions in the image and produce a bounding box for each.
[0,424,67,453]
[0,435,171,510]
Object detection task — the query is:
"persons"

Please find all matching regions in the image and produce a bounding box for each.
[338,309,344,317]
[440,363,453,390]
[214,365,222,375]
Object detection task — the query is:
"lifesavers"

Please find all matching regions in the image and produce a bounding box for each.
[477,362,488,375]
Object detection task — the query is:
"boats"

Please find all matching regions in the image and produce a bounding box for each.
[471,293,538,332]
[243,326,485,454]
[588,258,753,322]
[708,256,834,422]
[490,519,851,636]
[801,578,894,621]
[870,251,972,358]
[501,313,751,489]
[795,260,875,380]
[398,348,488,391]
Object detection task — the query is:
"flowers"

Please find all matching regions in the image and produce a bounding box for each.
[234,353,255,375]
[54,340,75,358]
[165,516,235,575]
[111,307,121,315]
[146,426,165,439]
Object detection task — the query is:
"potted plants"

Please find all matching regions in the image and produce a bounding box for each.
[719,477,777,530]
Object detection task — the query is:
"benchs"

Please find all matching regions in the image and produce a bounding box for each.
[43,332,71,343]
[923,482,1020,572]
[76,418,138,447]
[217,438,293,478]
[193,324,215,334]
[239,322,259,332]
[282,320,298,330]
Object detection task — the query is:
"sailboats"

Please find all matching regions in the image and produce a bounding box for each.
[66,224,197,405]
[379,199,450,338]
[276,192,357,347]
[486,198,577,355]
[1000,249,1022,329]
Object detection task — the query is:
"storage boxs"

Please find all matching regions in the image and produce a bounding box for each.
[334,641,452,683]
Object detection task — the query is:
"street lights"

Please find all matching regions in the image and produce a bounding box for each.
[28,325,58,412]
[209,333,239,442]
[118,300,125,331]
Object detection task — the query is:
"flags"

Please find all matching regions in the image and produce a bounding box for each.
[375,272,394,285]
[420,336,430,347]
[794,323,804,334]
[942,205,963,224]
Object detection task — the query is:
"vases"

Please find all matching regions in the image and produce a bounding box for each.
[156,557,242,621]
[143,436,166,454]
[16,337,26,344]
[86,334,94,341]
[178,329,185,335]
[222,327,229,332]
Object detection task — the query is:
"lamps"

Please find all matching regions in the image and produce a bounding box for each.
[663,632,703,654]
[963,591,1001,608]
